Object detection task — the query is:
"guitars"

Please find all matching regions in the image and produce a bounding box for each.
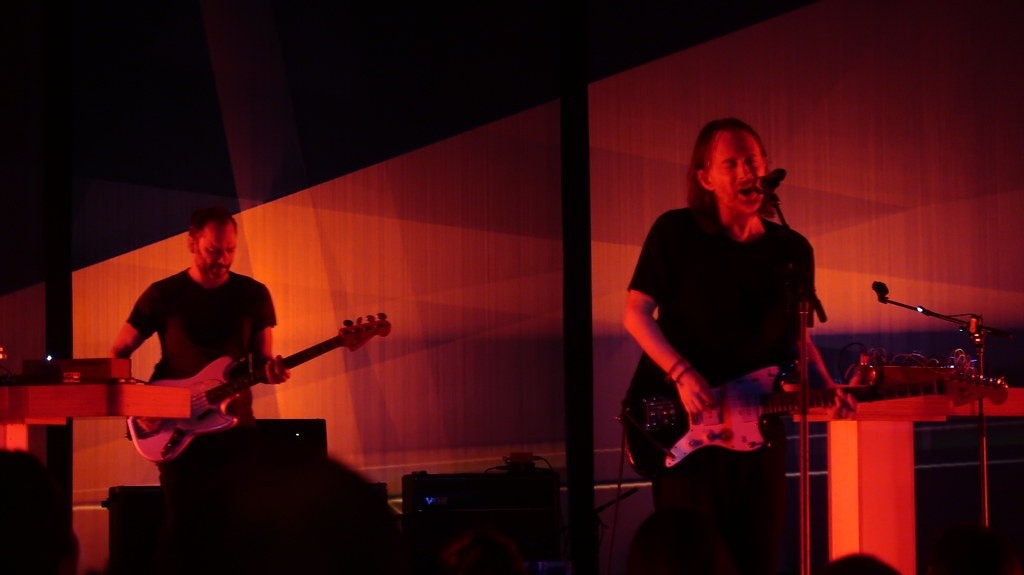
[123,304,398,467]
[659,356,1014,475]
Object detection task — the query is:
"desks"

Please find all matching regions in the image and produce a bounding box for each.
[0,383,191,524]
[827,388,1024,575]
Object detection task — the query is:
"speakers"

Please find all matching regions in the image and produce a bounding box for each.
[401,472,564,575]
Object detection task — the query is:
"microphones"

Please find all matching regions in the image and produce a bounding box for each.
[753,168,786,194]
